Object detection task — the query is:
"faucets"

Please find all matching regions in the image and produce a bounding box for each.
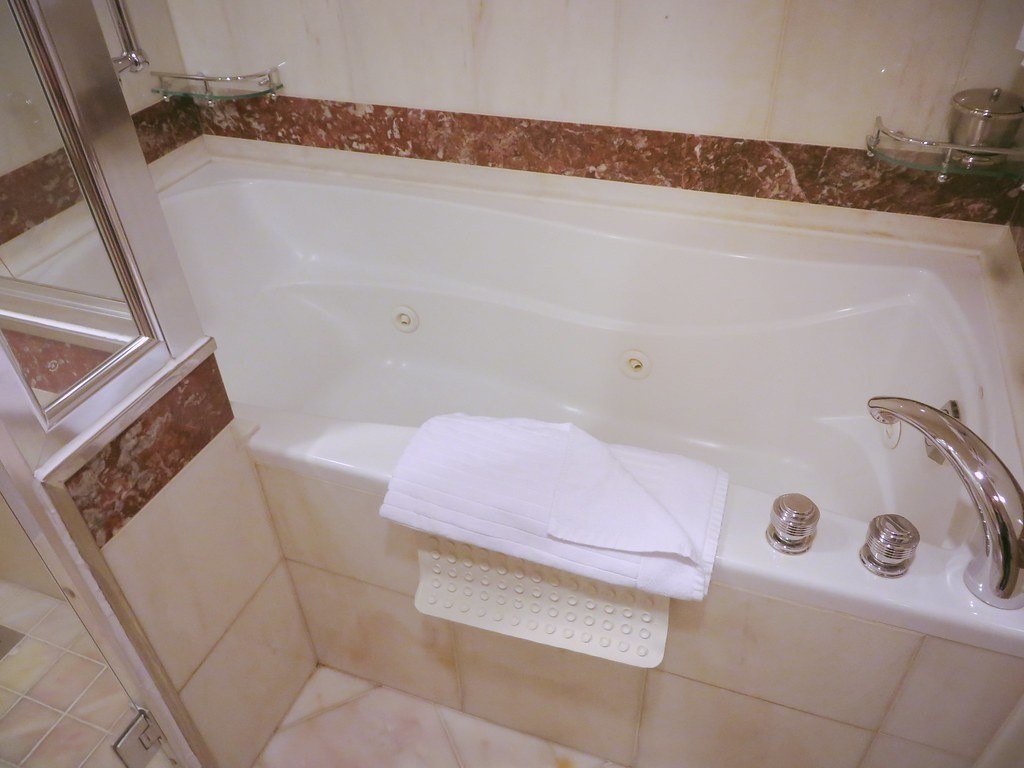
[865,395,1023,613]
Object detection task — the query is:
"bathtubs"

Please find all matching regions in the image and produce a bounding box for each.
[11,154,1024,767]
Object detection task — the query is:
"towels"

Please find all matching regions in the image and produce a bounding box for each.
[377,410,732,603]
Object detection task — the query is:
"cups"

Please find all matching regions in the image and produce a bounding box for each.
[949,86,1024,156]
